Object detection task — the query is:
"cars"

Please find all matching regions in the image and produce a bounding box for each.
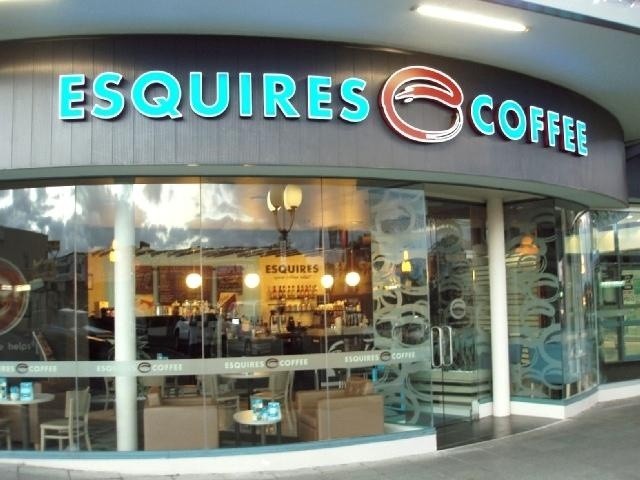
[42,301,117,353]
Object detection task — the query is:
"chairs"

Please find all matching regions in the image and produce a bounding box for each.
[0,351,390,452]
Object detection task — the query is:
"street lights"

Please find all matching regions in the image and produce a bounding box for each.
[265,182,303,257]
[320,271,366,303]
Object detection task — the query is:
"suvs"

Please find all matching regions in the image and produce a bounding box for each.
[174,300,271,357]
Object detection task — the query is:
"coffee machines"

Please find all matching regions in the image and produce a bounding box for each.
[268,306,287,334]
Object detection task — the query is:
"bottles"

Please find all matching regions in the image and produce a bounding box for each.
[289,316,295,328]
[320,299,362,326]
[267,283,320,311]
[169,297,208,317]
